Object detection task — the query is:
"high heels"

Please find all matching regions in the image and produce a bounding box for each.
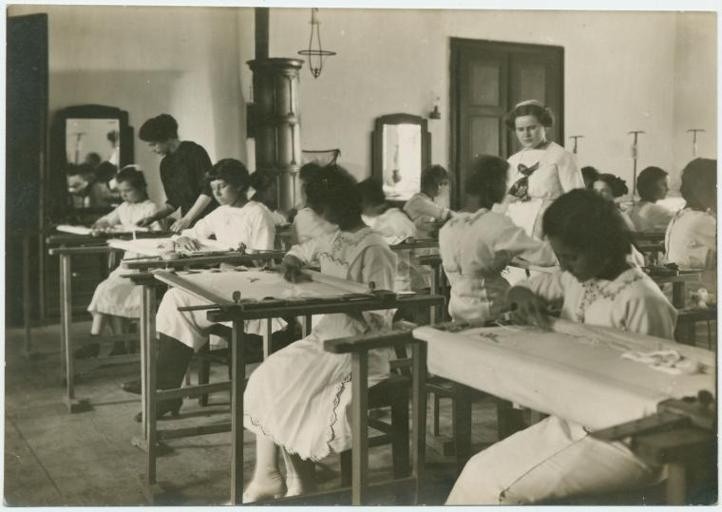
[133,396,183,422]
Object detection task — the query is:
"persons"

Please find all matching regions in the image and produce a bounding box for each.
[437,99,718,504]
[84,115,460,505]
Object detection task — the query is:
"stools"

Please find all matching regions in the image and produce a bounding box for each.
[38,207,717,505]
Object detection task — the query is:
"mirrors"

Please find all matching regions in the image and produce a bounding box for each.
[48,103,138,215]
[366,112,436,212]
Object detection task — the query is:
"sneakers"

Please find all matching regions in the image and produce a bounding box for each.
[122,377,181,395]
